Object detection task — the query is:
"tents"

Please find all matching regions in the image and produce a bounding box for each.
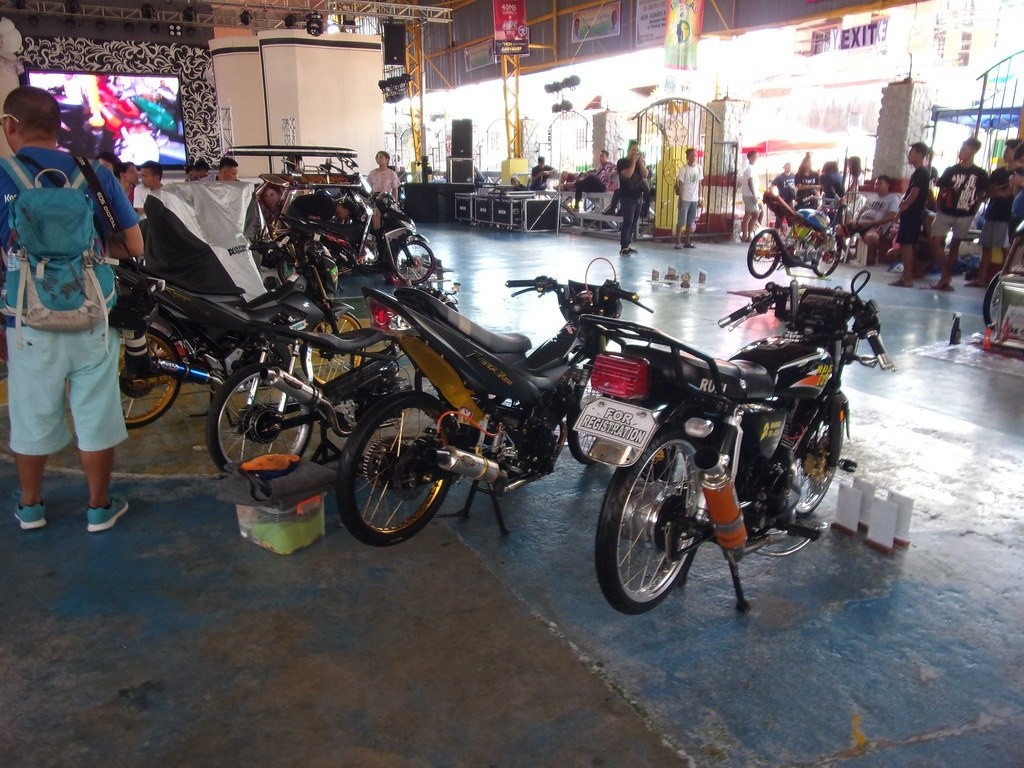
[697,110,837,191]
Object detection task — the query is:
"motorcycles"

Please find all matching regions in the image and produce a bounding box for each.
[745,191,844,279]
[572,269,896,614]
[337,258,654,552]
[108,184,459,480]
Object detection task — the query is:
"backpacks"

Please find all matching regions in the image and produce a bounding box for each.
[0,156,118,331]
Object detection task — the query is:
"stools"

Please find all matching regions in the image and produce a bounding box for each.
[844,232,878,266]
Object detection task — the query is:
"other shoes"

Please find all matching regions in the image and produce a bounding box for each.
[739,235,747,241]
[675,244,682,249]
[888,280,913,287]
[602,206,615,214]
[620,249,631,255]
[628,248,638,254]
[931,284,954,292]
[684,242,694,248]
[964,279,988,287]
[616,208,623,217]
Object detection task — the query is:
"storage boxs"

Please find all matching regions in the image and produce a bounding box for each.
[455,192,559,234]
[235,491,328,556]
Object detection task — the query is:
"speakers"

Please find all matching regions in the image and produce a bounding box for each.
[452,119,472,158]
[383,22,405,65]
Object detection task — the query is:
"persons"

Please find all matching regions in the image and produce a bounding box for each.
[964,139,1024,288]
[511,176,530,191]
[367,150,399,229]
[554,149,617,211]
[133,161,164,220]
[262,184,283,218]
[930,138,989,290]
[617,140,648,257]
[836,175,900,266]
[529,157,558,190]
[0,86,144,531]
[769,156,865,234]
[602,189,623,216]
[742,150,759,244]
[96,152,139,205]
[286,193,355,262]
[186,156,239,182]
[675,149,704,249]
[891,143,930,287]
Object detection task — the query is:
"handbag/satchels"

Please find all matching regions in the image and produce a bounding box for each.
[638,177,650,192]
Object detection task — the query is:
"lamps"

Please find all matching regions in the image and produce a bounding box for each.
[379,74,413,104]
[14,0,324,36]
[544,77,581,112]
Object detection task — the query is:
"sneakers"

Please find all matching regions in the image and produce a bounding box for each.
[87,493,128,532]
[14,497,46,530]
